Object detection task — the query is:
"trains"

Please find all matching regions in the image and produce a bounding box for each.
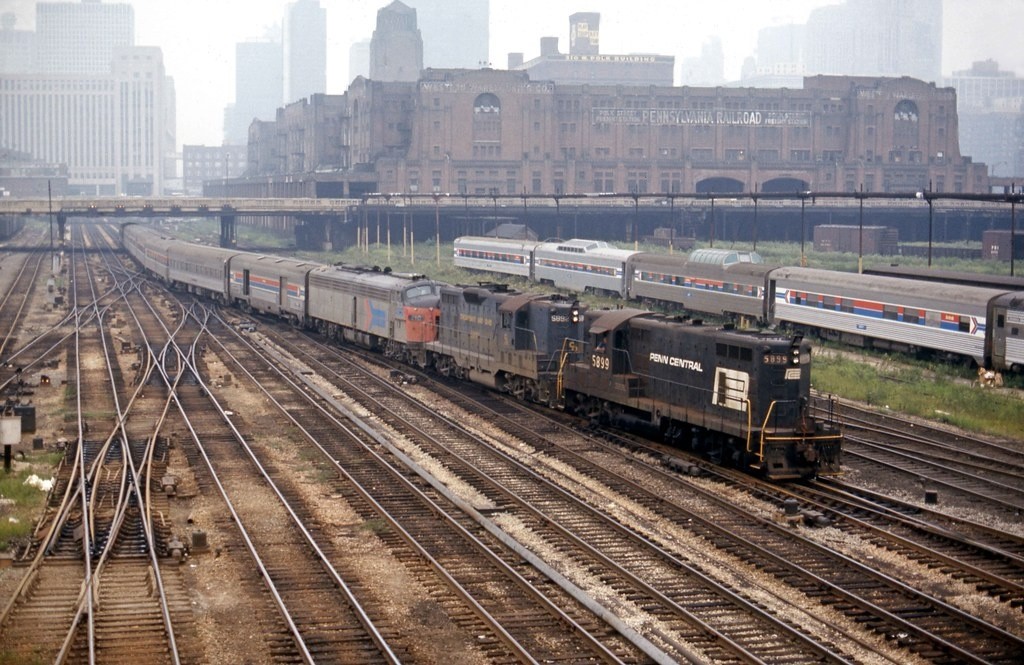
[117,221,846,483]
[452,233,1024,377]
